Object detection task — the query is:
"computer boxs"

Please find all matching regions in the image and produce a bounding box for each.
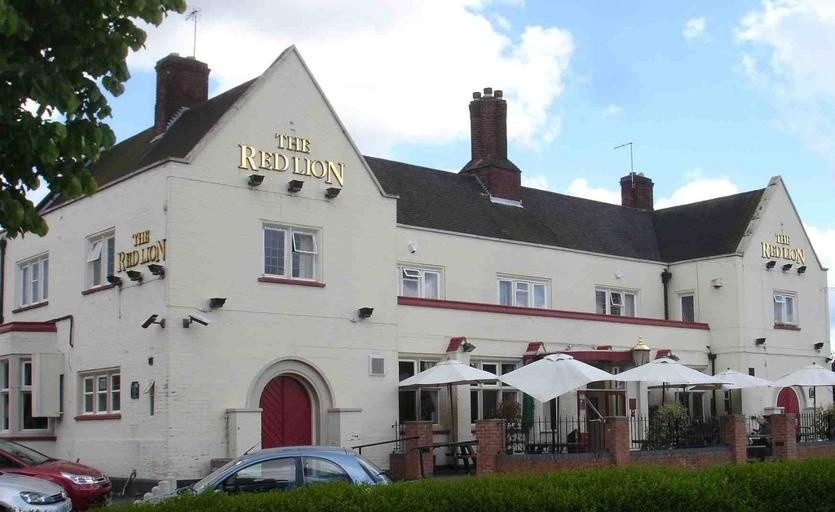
[409,243,416,253]
[141,313,158,328]
[189,313,211,327]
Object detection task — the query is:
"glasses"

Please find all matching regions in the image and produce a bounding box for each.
[460,338,476,352]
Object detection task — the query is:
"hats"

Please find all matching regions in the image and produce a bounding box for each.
[132,444,392,508]
[0,437,113,512]
[0,470,73,512]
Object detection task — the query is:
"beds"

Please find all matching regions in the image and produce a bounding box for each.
[690,367,772,412]
[398,352,500,449]
[492,353,612,453]
[768,362,835,433]
[593,355,734,408]
